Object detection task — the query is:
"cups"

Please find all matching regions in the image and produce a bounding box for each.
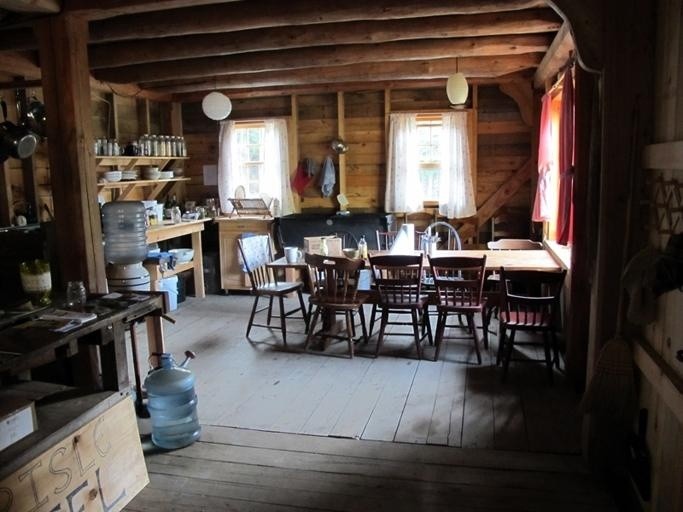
[67,281,89,307]
[283,246,303,265]
[19,268,53,306]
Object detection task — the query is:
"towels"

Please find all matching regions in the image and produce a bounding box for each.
[235,237,272,272]
[321,157,336,196]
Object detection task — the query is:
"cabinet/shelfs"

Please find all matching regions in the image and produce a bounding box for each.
[214,216,274,294]
[102,217,212,299]
[0,291,170,512]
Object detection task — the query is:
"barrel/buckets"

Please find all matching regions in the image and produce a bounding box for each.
[178,274,186,302]
[145,354,202,451]
[102,200,149,266]
[158,276,180,313]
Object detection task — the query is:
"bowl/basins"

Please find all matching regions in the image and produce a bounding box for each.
[168,249,194,264]
[144,168,161,180]
[104,170,123,183]
[343,247,359,259]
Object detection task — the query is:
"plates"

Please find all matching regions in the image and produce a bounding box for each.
[122,170,138,181]
[160,171,174,179]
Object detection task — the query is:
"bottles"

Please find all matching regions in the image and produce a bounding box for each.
[137,133,186,157]
[358,234,368,261]
[429,236,436,254]
[273,198,280,217]
[170,193,178,207]
[95,136,122,156]
[164,194,172,219]
[419,233,428,259]
[65,280,86,308]
[318,238,328,256]
[148,207,158,226]
[170,205,181,224]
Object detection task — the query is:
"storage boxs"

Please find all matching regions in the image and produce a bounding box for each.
[0,397,37,451]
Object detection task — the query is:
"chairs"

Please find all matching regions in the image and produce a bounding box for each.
[368,253,433,359]
[433,209,480,250]
[387,213,433,249]
[305,253,367,358]
[305,231,359,333]
[418,221,465,328]
[427,254,486,365]
[487,239,543,331]
[496,267,568,376]
[369,229,429,338]
[491,215,537,241]
[237,233,307,344]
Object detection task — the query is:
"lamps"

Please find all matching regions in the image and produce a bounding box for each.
[202,76,232,122]
[446,58,469,112]
